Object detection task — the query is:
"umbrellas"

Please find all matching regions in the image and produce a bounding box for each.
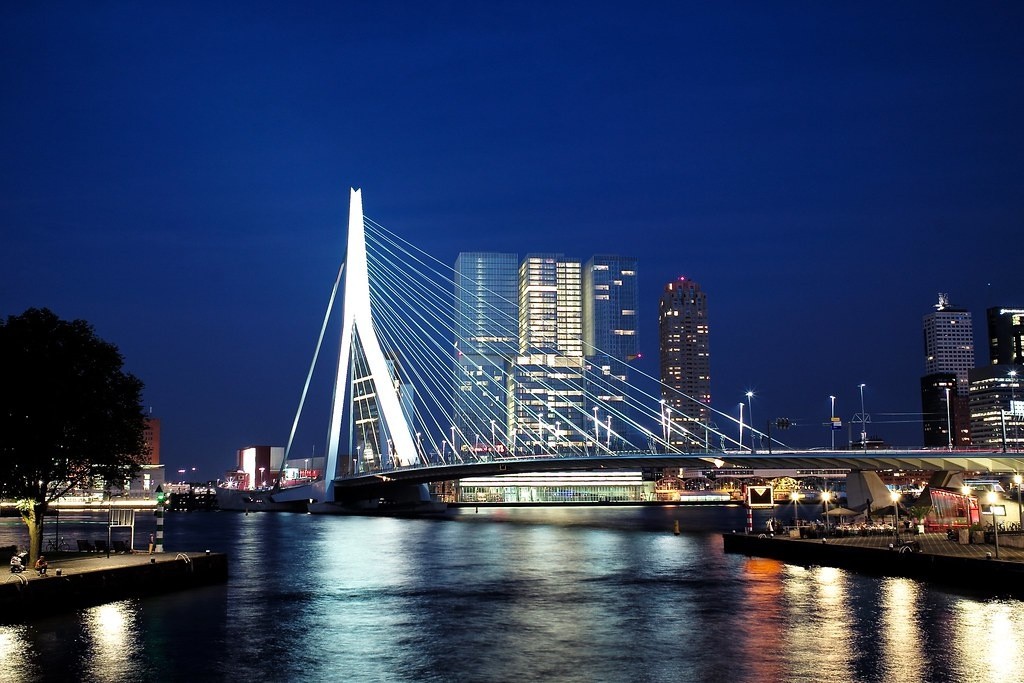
[820,507,861,526]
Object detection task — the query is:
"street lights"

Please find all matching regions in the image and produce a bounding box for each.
[442,441,446,458]
[1016,476,1023,531]
[858,384,866,450]
[792,494,798,520]
[739,403,745,451]
[962,486,970,528]
[988,492,1000,558]
[746,392,756,450]
[660,399,673,453]
[490,420,496,454]
[946,389,951,444]
[830,396,836,451]
[593,407,598,441]
[108,494,122,546]
[823,493,830,529]
[416,433,421,457]
[451,426,455,448]
[1008,371,1019,454]
[892,494,899,535]
[556,422,561,449]
[537,413,543,453]
[607,415,612,449]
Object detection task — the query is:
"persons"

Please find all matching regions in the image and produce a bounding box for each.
[765,516,926,539]
[34,556,48,577]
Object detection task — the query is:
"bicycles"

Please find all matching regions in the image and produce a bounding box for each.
[46,537,70,552]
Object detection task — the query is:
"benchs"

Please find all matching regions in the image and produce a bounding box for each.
[113,540,131,553]
[77,540,94,553]
[95,540,112,553]
[33,562,53,576]
